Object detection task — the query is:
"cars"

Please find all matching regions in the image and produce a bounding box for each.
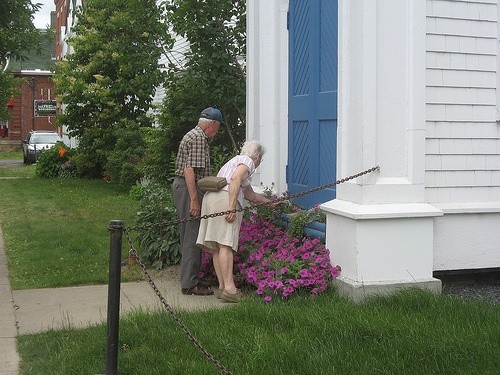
[22,130,64,164]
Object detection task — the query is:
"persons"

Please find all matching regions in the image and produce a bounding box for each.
[195,140,283,303]
[171,108,226,295]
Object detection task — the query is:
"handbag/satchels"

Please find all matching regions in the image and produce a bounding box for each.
[197,177,227,192]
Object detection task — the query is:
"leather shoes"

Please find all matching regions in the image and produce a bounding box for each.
[217,288,246,302]
[181,282,214,295]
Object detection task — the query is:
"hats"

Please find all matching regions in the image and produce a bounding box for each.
[199,107,227,130]
